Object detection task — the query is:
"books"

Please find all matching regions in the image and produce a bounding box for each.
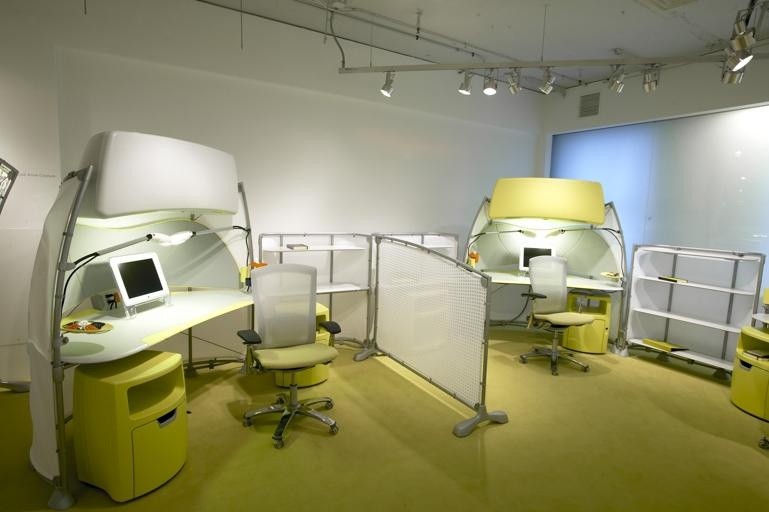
[658,275,688,283]
[287,243,308,251]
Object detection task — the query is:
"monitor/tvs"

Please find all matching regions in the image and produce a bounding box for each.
[106,251,175,320]
[518,243,556,277]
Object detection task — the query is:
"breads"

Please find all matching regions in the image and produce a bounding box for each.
[64,321,105,332]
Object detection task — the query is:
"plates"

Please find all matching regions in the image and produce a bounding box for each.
[601,272,623,279]
[62,321,113,332]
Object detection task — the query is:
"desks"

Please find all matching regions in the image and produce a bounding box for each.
[481,267,624,293]
[750,313,769,324]
[55,288,253,367]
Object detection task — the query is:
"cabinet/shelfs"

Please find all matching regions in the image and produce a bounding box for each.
[275,299,331,388]
[386,230,460,267]
[257,232,372,347]
[72,349,189,503]
[729,324,769,422]
[622,243,767,376]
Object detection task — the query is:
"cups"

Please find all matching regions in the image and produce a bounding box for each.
[517,271,526,281]
[124,304,137,320]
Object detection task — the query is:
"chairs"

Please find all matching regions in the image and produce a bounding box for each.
[237,256,341,448]
[520,254,597,375]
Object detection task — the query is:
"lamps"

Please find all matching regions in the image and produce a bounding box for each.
[58,231,172,271]
[336,60,665,97]
[719,11,763,85]
[546,227,619,239]
[469,229,536,238]
[172,225,250,246]
